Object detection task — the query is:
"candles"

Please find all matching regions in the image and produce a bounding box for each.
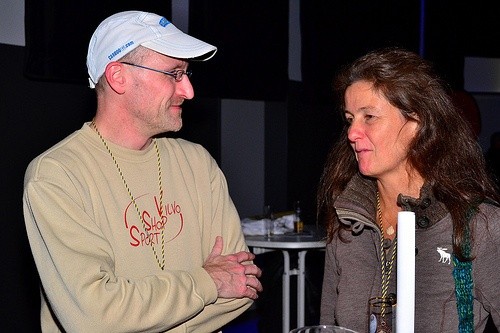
[395,210,416,333]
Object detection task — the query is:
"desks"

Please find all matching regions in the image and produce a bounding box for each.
[245,224,328,333]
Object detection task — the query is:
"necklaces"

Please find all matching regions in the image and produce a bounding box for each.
[380,212,397,235]
[92,116,165,272]
[377,191,398,333]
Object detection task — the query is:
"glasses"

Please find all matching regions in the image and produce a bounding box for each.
[119,60,193,82]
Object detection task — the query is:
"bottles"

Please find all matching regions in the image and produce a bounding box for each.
[294,201,303,232]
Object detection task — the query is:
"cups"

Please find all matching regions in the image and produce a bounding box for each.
[287,324,356,333]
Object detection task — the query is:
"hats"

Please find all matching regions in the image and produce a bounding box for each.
[86,10,218,90]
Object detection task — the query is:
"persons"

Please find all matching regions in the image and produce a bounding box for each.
[318,49,500,333]
[23,11,262,333]
